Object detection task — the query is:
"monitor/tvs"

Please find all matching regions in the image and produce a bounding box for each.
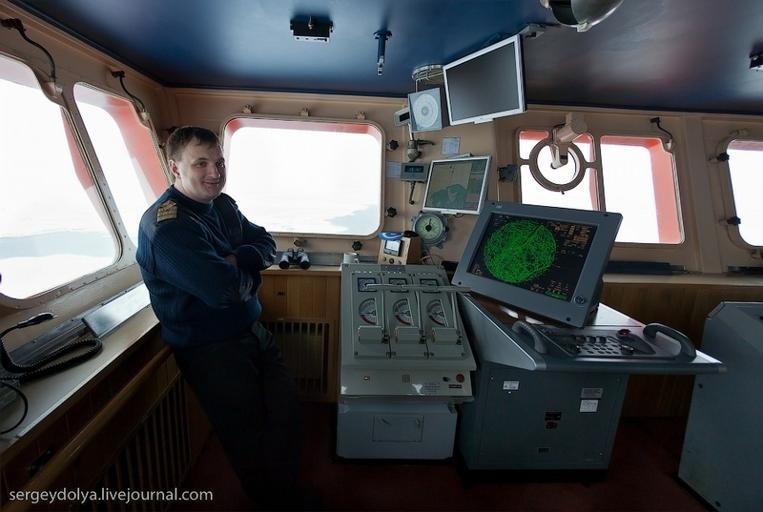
[451,200,623,328]
[442,33,527,126]
[422,155,492,216]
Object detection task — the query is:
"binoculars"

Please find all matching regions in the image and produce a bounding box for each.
[278,247,310,270]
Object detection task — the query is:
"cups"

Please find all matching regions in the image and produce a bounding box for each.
[343,251,359,263]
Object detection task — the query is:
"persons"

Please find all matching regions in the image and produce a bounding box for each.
[132,122,323,511]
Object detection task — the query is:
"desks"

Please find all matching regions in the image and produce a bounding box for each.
[0,287,193,512]
[256,251,341,403]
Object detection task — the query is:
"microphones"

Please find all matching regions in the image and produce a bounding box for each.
[0,312,54,339]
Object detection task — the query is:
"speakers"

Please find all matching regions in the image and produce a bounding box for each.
[408,88,450,132]
